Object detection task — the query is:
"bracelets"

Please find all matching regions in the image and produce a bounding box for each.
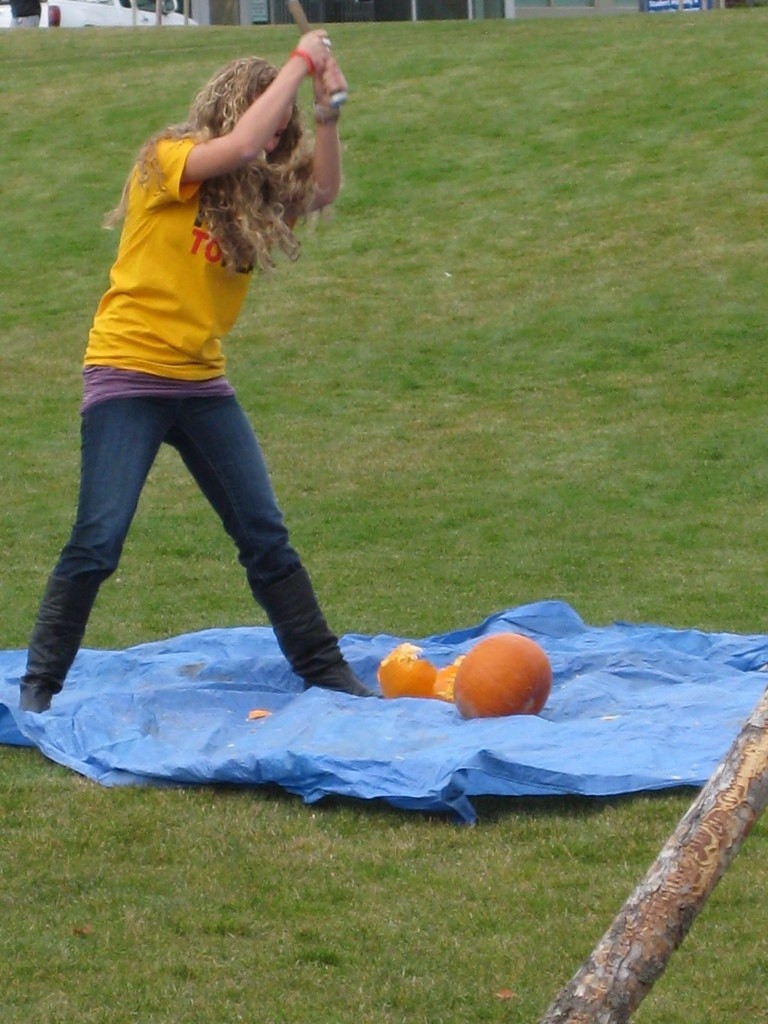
[292,51,313,70]
[313,101,339,124]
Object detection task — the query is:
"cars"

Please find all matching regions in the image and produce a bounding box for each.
[0,0,201,30]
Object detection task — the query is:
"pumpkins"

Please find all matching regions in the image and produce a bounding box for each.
[455,633,552,716]
[377,642,458,700]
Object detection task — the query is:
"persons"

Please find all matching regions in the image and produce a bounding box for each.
[18,30,388,713]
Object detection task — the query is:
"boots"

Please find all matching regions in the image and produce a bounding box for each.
[19,574,99,714]
[252,566,384,699]
